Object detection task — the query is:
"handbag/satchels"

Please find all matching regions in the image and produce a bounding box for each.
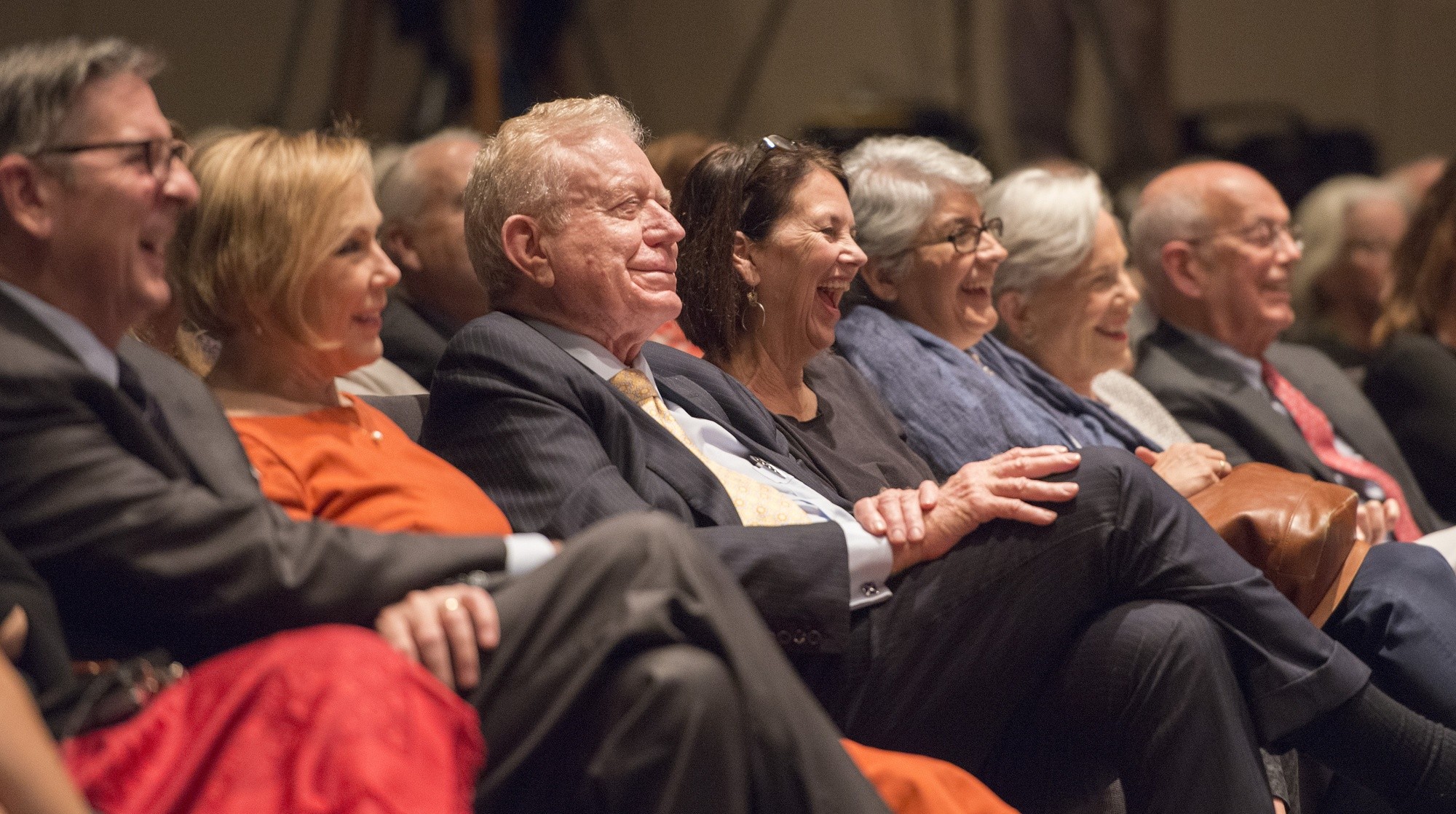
[1187,460,1360,616]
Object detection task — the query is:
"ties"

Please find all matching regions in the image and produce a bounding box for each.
[609,368,811,526]
[116,362,214,488]
[1263,359,1424,542]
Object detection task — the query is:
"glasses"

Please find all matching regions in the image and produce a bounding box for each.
[28,137,190,183]
[888,216,1004,260]
[1202,222,1304,251]
[738,134,800,190]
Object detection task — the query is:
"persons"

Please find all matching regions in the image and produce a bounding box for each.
[0,37,1456,814]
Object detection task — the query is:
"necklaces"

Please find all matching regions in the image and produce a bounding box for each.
[970,353,995,377]
[317,401,384,442]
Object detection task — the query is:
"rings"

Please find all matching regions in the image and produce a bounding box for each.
[440,598,460,612]
[1217,460,1225,476]
